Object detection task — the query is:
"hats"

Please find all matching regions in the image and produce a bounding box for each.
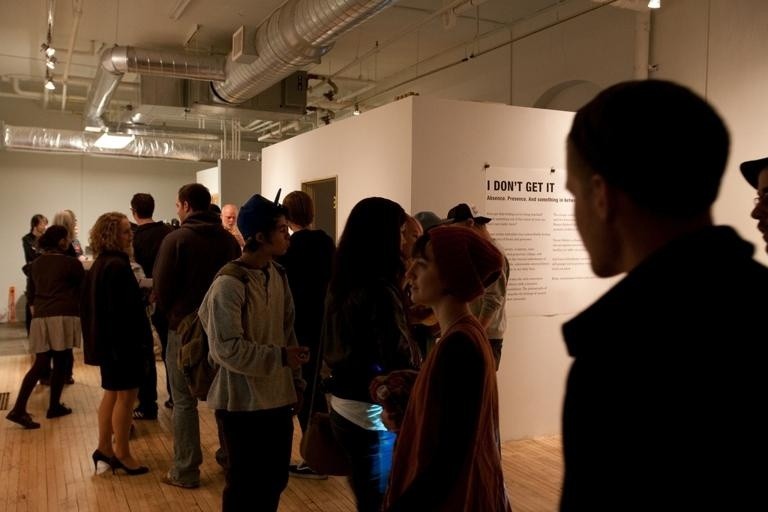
[739,156,768,188]
[446,203,492,226]
[430,224,503,305]
[237,189,285,242]
[416,210,454,230]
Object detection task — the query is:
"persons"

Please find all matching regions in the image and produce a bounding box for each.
[218,203,246,252]
[275,192,348,480]
[442,203,512,372]
[6,225,87,429]
[177,193,313,511]
[54,209,83,257]
[124,193,180,420]
[21,214,48,335]
[558,78,768,512]
[149,183,242,490]
[739,155,768,258]
[323,197,407,512]
[82,212,150,477]
[370,226,513,511]
[407,212,443,360]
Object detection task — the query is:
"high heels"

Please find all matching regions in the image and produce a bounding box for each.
[92,449,116,469]
[108,453,150,475]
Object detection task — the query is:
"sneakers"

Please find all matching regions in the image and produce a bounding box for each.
[160,470,200,490]
[6,408,40,430]
[165,399,174,408]
[46,404,72,418]
[289,462,328,480]
[131,408,157,420]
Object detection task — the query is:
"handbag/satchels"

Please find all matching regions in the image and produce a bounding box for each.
[298,293,349,477]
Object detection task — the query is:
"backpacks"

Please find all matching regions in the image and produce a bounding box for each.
[166,260,293,400]
